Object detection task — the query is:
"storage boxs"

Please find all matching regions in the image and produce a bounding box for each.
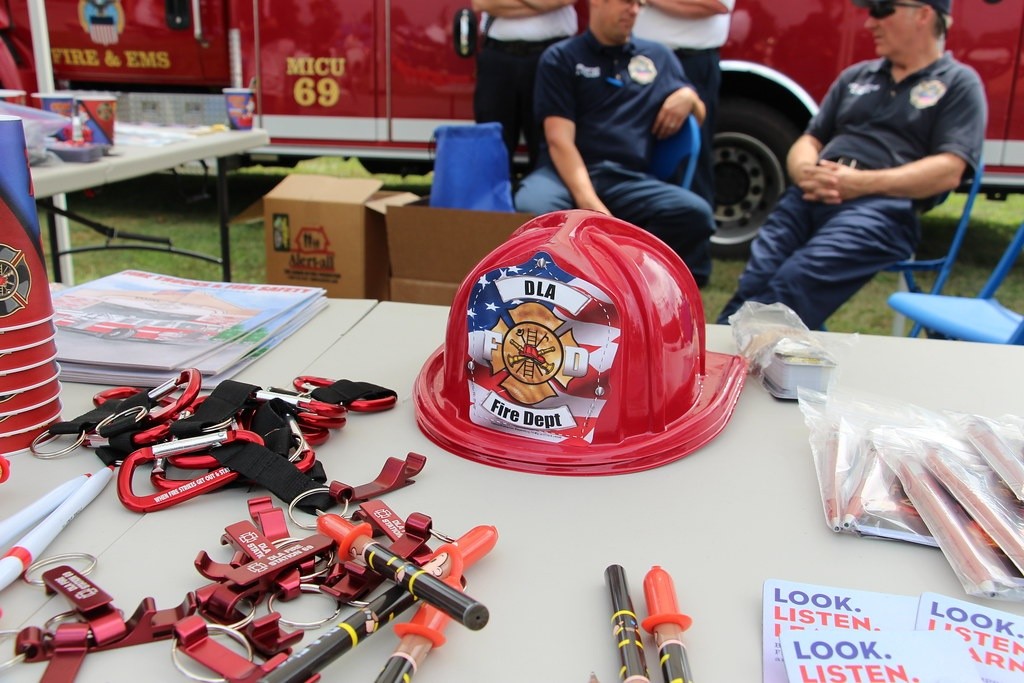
[260,168,420,299]
[386,195,537,307]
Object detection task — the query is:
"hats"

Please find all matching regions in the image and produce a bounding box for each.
[852,0,951,14]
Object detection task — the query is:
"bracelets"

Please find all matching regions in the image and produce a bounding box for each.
[640,0,645,5]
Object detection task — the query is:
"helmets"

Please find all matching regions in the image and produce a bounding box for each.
[413,208,752,476]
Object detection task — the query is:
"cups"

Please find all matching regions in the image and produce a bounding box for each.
[0,116,63,458]
[0,90,28,109]
[32,90,75,142]
[222,87,256,130]
[75,94,117,154]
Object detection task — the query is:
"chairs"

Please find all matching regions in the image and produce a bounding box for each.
[886,222,1024,345]
[641,112,702,189]
[426,123,516,211]
[876,156,984,340]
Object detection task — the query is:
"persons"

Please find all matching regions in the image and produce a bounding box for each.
[716,0,988,332]
[515,0,717,290]
[631,0,737,212]
[471,0,578,194]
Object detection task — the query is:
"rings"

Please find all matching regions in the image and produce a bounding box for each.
[669,123,675,127]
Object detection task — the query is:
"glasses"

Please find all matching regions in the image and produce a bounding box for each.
[869,2,923,18]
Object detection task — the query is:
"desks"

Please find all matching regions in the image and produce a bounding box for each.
[20,125,272,285]
[0,301,1024,683]
